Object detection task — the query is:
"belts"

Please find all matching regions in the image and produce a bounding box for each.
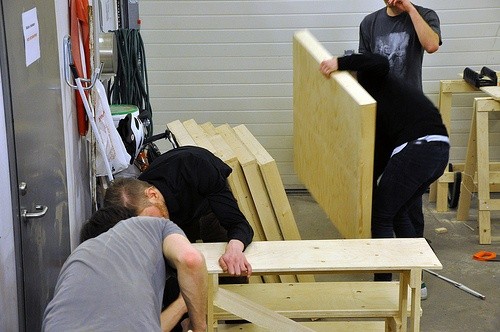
[409,140,426,145]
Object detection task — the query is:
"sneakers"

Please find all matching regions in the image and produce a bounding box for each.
[421,281,427,300]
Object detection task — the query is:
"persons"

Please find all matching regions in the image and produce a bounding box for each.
[320,51,450,300]
[40,205,209,332]
[359,0,443,238]
[104,145,254,332]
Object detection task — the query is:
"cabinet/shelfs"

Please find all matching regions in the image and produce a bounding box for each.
[191,238,442,332]
